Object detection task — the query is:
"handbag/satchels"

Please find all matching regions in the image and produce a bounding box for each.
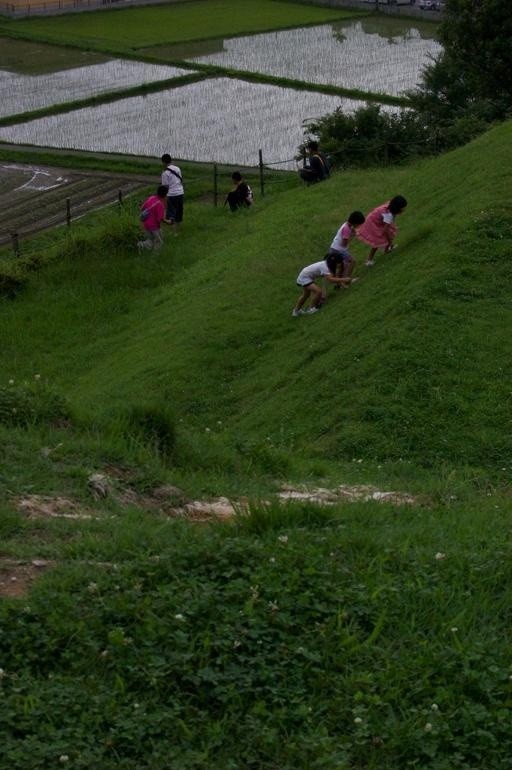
[138,208,151,220]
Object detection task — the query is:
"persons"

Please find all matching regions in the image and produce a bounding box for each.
[329,210,365,291]
[291,252,352,317]
[430,0,437,11]
[223,172,254,217]
[298,142,330,188]
[137,185,172,257]
[355,195,408,267]
[161,153,184,237]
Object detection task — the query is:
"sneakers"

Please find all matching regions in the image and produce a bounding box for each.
[308,306,319,313]
[292,308,306,317]
